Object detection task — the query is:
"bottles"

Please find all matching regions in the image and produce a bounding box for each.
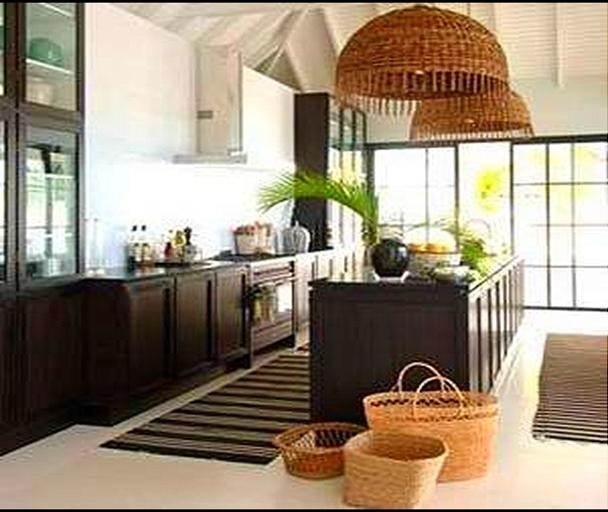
[128,223,200,267]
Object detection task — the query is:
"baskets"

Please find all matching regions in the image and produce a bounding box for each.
[343,430,448,510]
[272,423,367,478]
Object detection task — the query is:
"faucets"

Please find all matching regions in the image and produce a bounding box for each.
[463,218,492,237]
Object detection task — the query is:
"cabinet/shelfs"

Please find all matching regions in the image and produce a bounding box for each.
[293,92,368,280]
[0,0,87,457]
[87,248,332,399]
[172,46,247,166]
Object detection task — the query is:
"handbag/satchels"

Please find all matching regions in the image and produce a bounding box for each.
[362,363,499,482]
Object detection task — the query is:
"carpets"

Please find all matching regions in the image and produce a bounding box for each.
[99,353,312,465]
[531,331,608,444]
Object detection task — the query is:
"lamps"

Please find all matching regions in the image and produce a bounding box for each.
[334,2,534,142]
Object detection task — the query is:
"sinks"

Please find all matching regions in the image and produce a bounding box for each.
[485,252,498,258]
